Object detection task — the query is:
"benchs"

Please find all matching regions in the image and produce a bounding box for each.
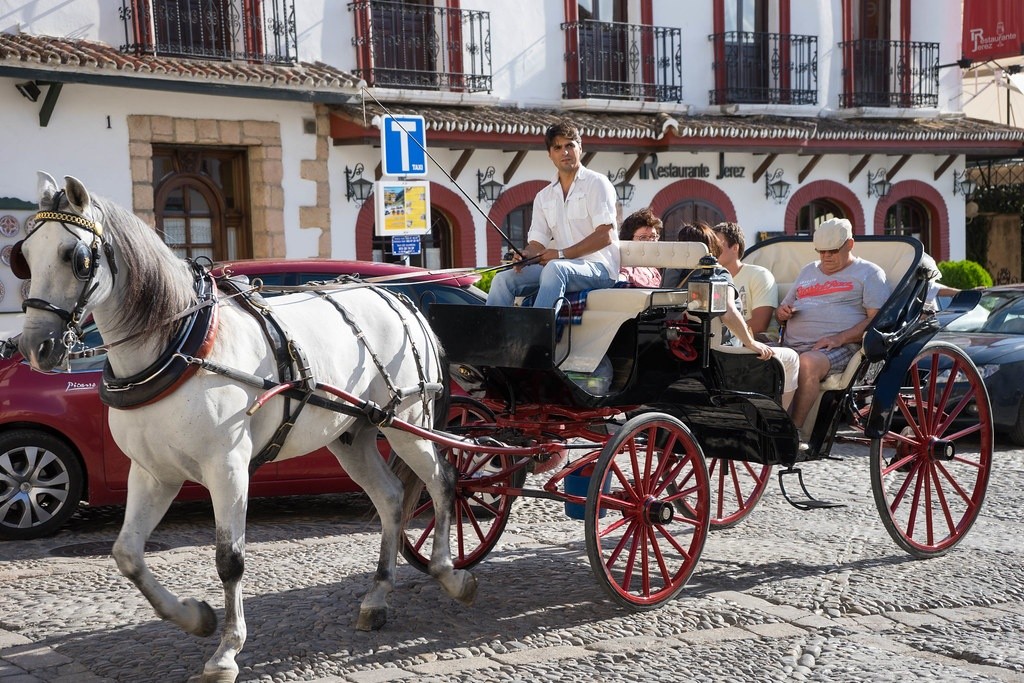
[761,283,883,394]
[709,317,786,399]
[513,240,713,327]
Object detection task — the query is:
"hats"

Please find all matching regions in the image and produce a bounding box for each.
[813,217,853,251]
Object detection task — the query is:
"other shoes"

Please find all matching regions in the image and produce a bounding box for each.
[796,427,804,444]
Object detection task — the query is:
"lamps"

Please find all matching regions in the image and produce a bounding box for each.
[953,170,978,197]
[344,162,373,211]
[15,79,49,102]
[931,58,974,71]
[765,167,791,205]
[868,168,892,200]
[608,168,636,207]
[476,166,504,207]
[967,199,979,225]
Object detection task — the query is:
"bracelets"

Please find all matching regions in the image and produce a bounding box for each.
[558,250,565,259]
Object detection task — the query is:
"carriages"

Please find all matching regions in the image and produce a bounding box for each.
[6,170,996,683]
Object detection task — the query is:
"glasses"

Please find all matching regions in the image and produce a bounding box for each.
[633,234,662,242]
[814,241,848,255]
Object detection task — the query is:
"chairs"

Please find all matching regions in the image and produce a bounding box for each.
[999,304,1024,334]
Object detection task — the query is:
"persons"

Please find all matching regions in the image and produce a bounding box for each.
[619,210,662,289]
[774,217,890,441]
[925,282,960,302]
[659,222,775,362]
[456,120,621,384]
[710,223,778,334]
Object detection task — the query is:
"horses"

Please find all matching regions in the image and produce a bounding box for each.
[19,171,477,683]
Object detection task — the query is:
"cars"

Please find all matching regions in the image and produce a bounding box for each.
[857,282,1024,450]
[0,257,526,543]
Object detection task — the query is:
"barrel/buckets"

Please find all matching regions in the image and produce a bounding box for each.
[564,437,613,520]
